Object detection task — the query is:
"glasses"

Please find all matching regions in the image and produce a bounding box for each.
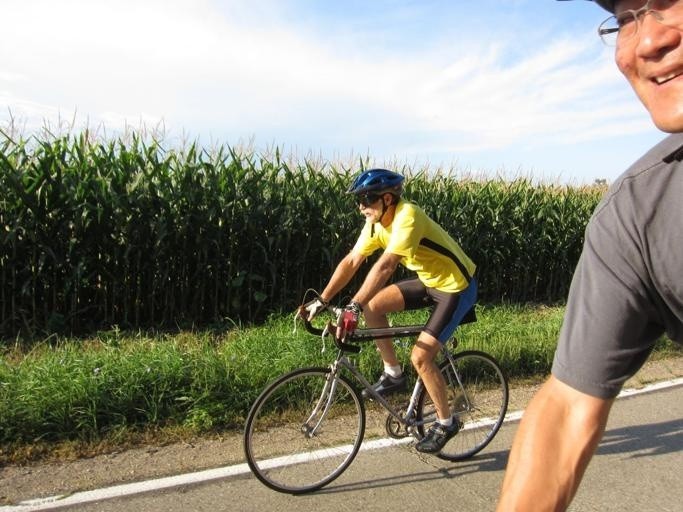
[355,194,383,207]
[596,1,681,48]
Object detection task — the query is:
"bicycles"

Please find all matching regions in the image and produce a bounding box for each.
[243,289,509,494]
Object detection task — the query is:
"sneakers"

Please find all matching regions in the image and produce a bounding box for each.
[414,415,461,454]
[361,367,406,400]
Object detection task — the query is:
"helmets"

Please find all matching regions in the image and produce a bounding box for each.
[345,169,403,200]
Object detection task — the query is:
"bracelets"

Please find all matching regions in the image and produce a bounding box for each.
[347,300,364,315]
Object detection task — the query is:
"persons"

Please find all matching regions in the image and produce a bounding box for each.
[292,168,479,454]
[497,1,682,512]
